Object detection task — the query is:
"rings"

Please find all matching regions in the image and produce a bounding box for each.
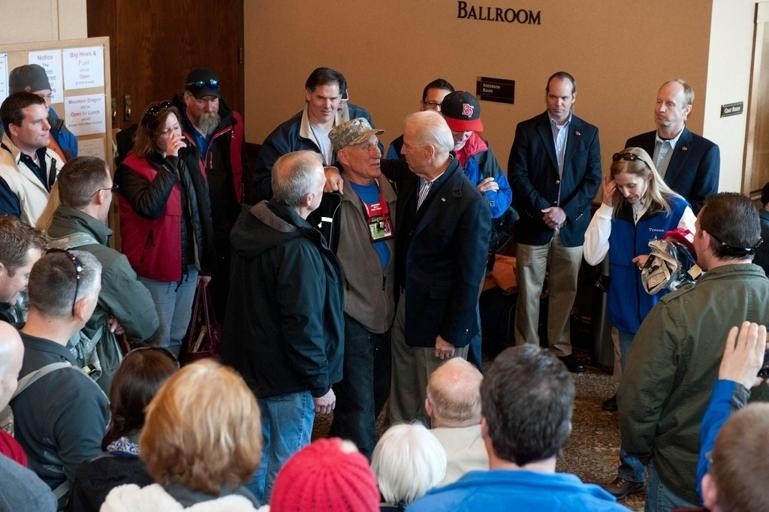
[639,266,642,270]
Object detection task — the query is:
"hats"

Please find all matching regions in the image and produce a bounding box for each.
[7,65,54,97]
[185,69,223,98]
[268,437,382,508]
[441,91,484,133]
[328,115,386,152]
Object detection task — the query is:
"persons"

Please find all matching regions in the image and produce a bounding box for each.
[321,111,491,410]
[384,77,462,176]
[366,418,449,508]
[248,65,380,210]
[699,399,769,511]
[0,92,73,233]
[262,438,379,511]
[404,342,633,512]
[604,76,722,409]
[111,98,213,366]
[11,155,160,400]
[220,148,347,430]
[0,211,50,330]
[1,63,79,164]
[159,69,244,241]
[101,358,266,511]
[69,346,192,508]
[424,354,492,487]
[315,116,402,410]
[694,318,769,510]
[506,71,605,373]
[14,247,118,512]
[403,89,512,371]
[581,145,702,382]
[0,321,58,511]
[615,192,769,511]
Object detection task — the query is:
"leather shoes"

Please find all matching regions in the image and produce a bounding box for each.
[603,395,619,411]
[558,355,587,372]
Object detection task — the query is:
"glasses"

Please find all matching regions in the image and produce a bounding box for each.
[144,101,171,121]
[696,231,765,255]
[40,247,86,320]
[613,150,648,165]
[86,181,122,200]
[186,79,220,90]
[422,101,444,108]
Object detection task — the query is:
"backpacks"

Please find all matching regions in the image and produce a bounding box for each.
[11,229,104,384]
